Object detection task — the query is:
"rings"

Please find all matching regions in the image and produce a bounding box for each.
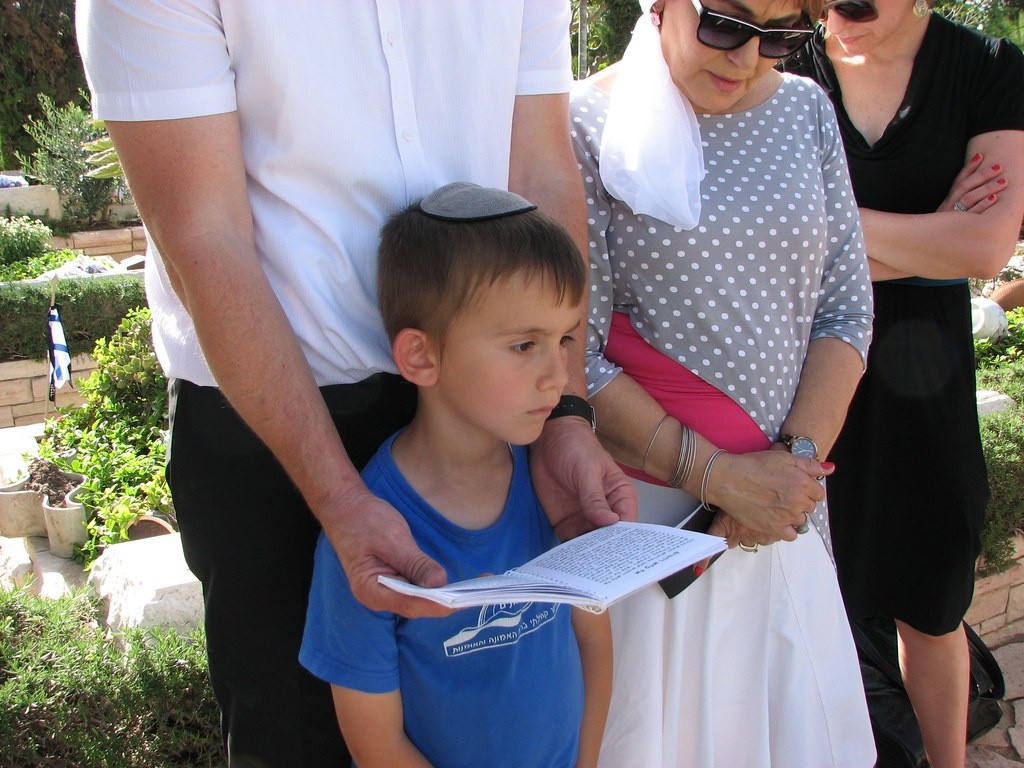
[796,512,810,534]
[739,542,757,552]
[953,203,967,211]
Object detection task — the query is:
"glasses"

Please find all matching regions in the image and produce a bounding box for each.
[690,1,824,58]
[827,1,881,24]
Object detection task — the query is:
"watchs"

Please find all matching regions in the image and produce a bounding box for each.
[776,435,824,480]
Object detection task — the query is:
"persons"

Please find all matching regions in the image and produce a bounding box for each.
[75,0,636,768]
[778,0,1024,768]
[300,182,613,768]
[571,0,874,768]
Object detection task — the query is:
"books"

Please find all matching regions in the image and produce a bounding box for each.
[377,520,729,613]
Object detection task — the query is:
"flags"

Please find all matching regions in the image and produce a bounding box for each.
[47,308,70,389]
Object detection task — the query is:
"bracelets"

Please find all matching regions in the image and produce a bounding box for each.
[546,394,596,431]
[667,423,696,490]
[640,415,670,470]
[701,449,726,510]
